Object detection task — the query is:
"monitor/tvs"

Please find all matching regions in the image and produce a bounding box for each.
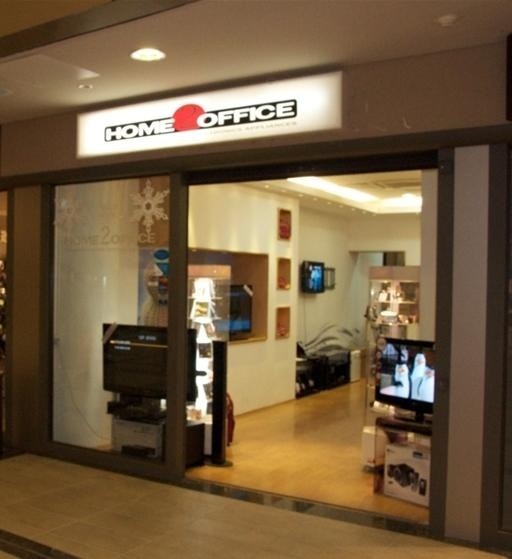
[102,323,197,399]
[301,261,324,293]
[375,337,435,424]
[217,284,253,340]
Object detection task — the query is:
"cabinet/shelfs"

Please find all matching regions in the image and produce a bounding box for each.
[366,266,422,393]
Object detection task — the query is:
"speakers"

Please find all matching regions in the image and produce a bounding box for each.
[212,340,225,468]
